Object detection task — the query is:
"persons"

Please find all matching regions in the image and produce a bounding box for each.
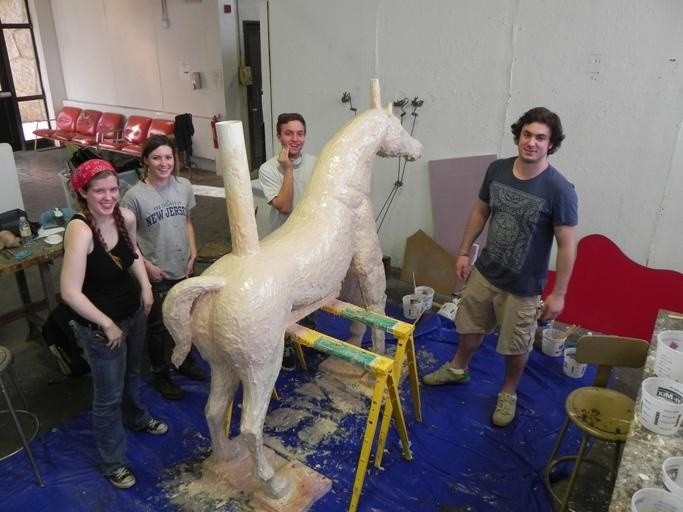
[423,107,577,425]
[120,136,205,400]
[59,158,167,489]
[258,113,317,372]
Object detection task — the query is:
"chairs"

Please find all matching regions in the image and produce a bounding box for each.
[541,331,650,512]
[32,104,185,187]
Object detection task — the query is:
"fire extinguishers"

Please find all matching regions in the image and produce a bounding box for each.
[210,113,222,149]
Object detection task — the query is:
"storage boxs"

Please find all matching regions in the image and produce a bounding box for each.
[57,168,142,214]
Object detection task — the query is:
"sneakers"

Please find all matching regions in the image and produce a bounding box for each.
[492,388,518,428]
[423,361,471,384]
[142,417,169,435]
[105,462,137,489]
[279,344,297,371]
[147,357,207,404]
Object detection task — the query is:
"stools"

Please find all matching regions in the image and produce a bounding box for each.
[0,347,50,491]
[194,239,231,265]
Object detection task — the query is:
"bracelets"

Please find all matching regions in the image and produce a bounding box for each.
[457,253,467,256]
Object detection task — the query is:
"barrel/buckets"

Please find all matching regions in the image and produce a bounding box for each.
[660,457,683,495]
[414,285,435,310]
[401,294,424,320]
[630,487,683,512]
[436,302,459,321]
[654,329,683,382]
[561,346,589,379]
[541,328,566,358]
[640,376,683,436]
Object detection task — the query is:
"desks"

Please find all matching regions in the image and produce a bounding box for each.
[0,225,70,387]
[606,307,682,512]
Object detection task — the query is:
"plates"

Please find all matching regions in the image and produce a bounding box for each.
[44,234,65,245]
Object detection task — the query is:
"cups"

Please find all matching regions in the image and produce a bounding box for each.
[541,327,588,379]
[401,282,461,320]
[631,328,683,511]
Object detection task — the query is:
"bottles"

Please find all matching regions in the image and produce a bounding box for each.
[17,216,34,248]
